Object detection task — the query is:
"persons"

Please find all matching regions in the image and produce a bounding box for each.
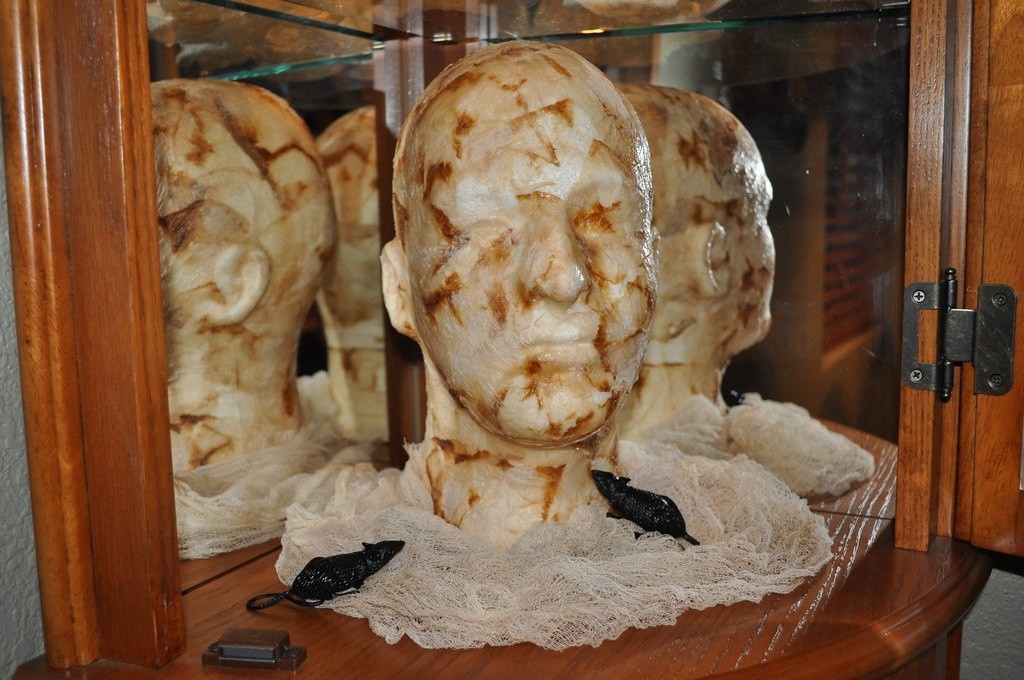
[383,42,659,527]
[296,109,389,438]
[149,78,337,490]
[611,79,776,435]
